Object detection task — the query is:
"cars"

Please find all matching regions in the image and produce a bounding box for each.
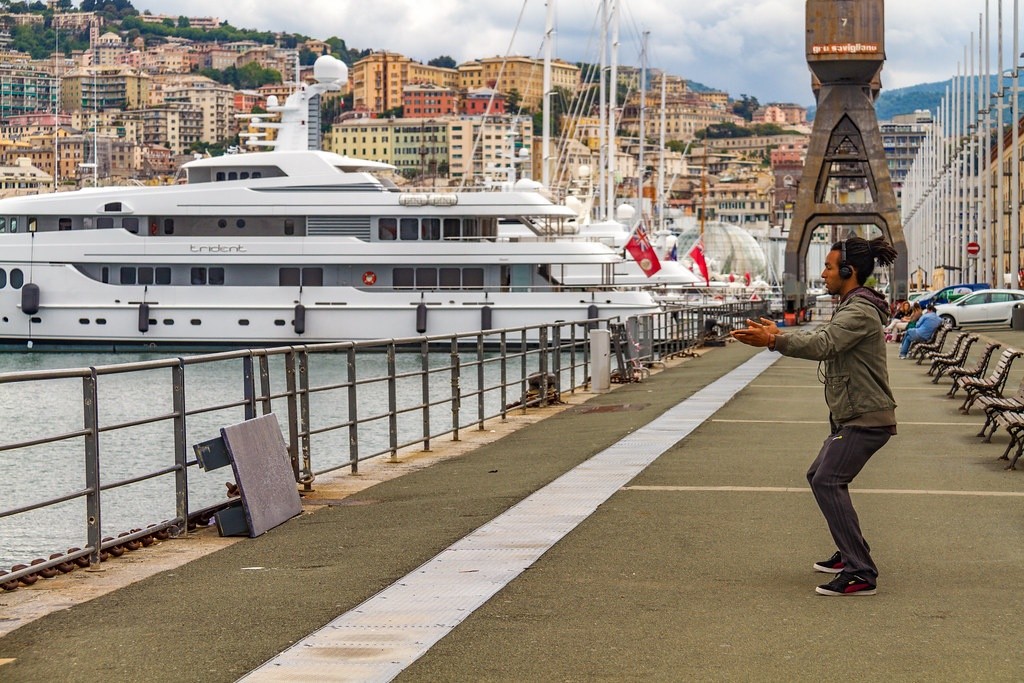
[914,282,991,312]
[922,288,1024,331]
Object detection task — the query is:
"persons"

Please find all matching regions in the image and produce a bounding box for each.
[883,302,922,343]
[889,302,913,321]
[898,303,943,360]
[150,222,158,235]
[729,235,900,596]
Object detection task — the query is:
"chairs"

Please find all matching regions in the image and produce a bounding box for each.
[957,346,1024,414]
[911,326,953,365]
[931,336,979,384]
[926,332,970,377]
[945,340,1002,399]
[976,373,1024,471]
[906,324,944,359]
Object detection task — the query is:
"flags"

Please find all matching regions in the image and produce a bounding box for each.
[689,236,709,287]
[625,224,661,279]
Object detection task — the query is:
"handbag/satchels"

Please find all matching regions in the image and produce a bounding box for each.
[884,334,901,343]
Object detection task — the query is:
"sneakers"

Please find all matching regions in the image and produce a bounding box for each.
[815,569,877,596]
[813,551,847,574]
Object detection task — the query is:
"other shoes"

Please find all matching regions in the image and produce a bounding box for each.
[883,328,892,334]
[887,340,896,344]
[897,355,906,359]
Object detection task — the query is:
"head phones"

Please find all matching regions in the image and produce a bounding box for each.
[839,238,852,279]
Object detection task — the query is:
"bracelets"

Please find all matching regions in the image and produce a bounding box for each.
[768,334,775,350]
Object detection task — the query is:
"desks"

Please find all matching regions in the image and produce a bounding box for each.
[194,412,303,539]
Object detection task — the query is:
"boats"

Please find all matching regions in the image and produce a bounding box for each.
[0,1,774,353]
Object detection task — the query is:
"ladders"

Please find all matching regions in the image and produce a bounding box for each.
[607,323,634,383]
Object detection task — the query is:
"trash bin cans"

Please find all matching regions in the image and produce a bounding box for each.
[589,329,611,394]
[1012,303,1024,332]
